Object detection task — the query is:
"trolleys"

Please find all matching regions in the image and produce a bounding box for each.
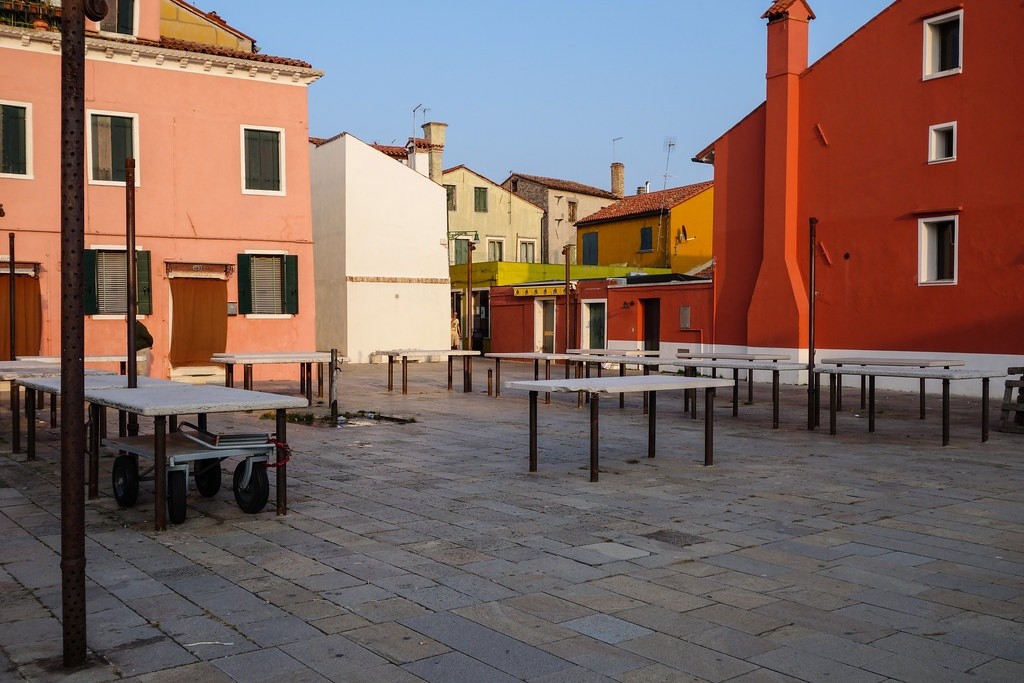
[100,420,280,526]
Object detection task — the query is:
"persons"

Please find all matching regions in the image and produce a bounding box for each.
[120,315,153,377]
[451,311,460,350]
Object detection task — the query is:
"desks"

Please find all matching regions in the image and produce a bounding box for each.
[376,350,1008,482]
[0,353,351,531]
